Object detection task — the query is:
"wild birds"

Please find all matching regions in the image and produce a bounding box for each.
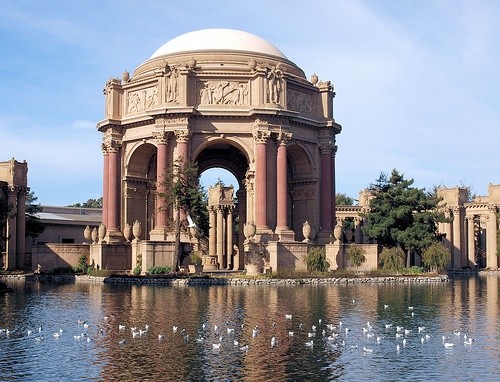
[0,300,476,358]
